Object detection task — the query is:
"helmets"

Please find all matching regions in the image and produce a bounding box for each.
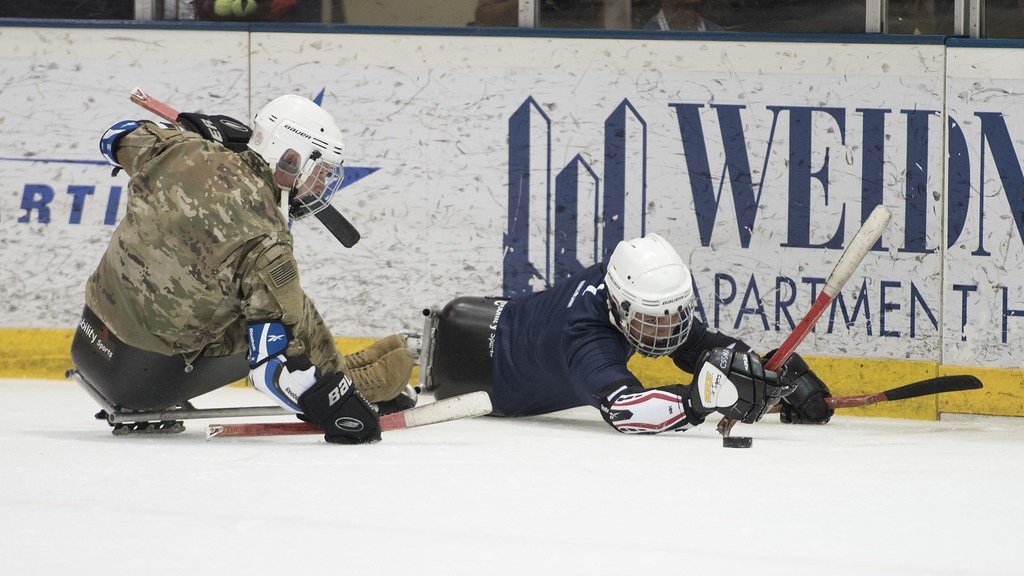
[246,94,345,218]
[604,232,694,358]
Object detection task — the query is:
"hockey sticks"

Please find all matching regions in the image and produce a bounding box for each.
[130,89,359,247]
[718,203,890,426]
[760,373,985,408]
[206,390,495,438]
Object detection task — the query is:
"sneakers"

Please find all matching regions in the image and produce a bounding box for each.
[344,334,408,370]
[346,347,414,404]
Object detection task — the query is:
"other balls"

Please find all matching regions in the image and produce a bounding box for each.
[722,436,753,448]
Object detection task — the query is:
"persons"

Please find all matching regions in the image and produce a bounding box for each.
[155,0,1024,39]
[71,96,412,445]
[434,234,836,435]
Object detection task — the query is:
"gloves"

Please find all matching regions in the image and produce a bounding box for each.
[175,112,253,153]
[298,372,382,444]
[761,348,834,425]
[682,347,798,424]
